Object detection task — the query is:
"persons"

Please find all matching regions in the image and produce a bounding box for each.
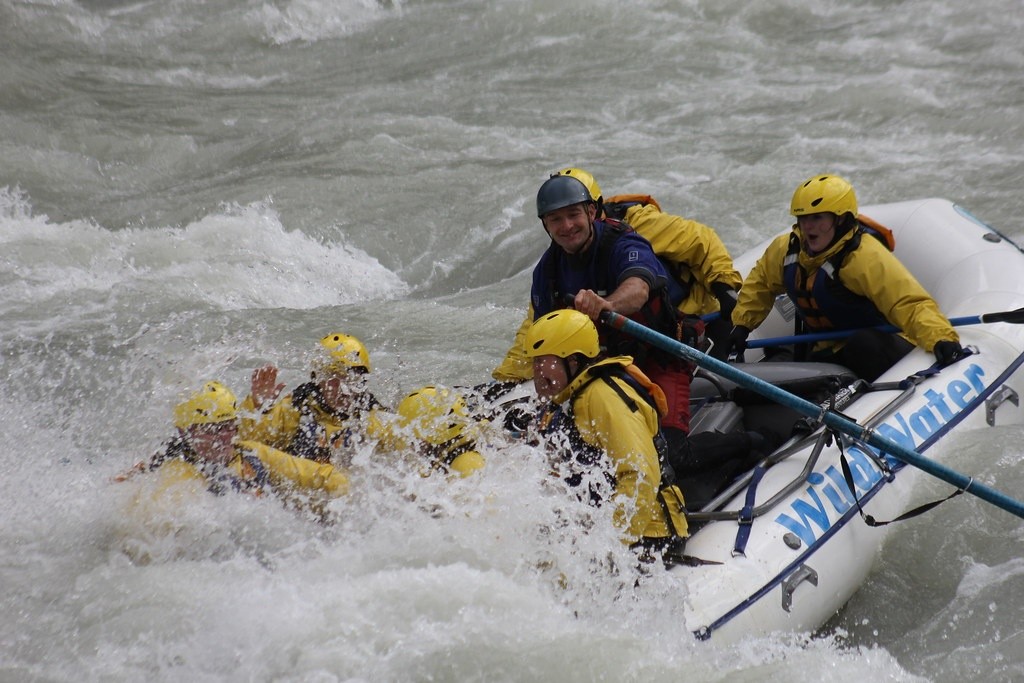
[521,310,690,570]
[727,174,960,369]
[489,168,742,445]
[148,332,487,501]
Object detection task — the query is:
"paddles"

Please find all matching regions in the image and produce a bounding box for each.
[743,306,1023,356]
[562,283,1023,518]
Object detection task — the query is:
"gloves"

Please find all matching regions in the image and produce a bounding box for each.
[934,339,962,365]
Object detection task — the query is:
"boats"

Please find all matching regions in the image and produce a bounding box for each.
[481,201,1024,646]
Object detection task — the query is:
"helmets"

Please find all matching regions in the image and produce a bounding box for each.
[405,387,468,443]
[790,173,859,221]
[524,308,601,359]
[317,332,370,376]
[536,169,603,219]
[173,381,239,426]
